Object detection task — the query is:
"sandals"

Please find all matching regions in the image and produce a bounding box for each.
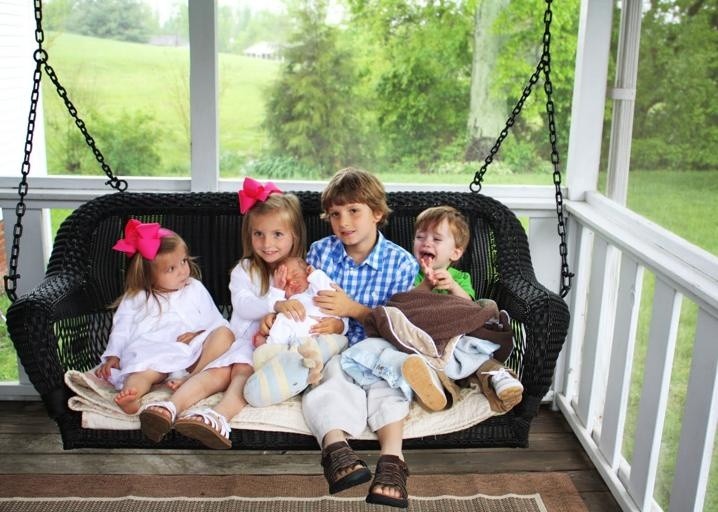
[173,408,232,449]
[139,401,176,443]
[365,455,409,508]
[321,441,371,495]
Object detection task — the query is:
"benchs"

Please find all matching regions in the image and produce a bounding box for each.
[6,190,571,450]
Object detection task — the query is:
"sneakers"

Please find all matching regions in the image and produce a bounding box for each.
[480,368,524,410]
[401,354,447,412]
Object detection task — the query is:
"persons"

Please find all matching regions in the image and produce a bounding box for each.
[399,205,525,413]
[94,219,236,414]
[252,256,349,372]
[300,167,422,509]
[135,177,307,451]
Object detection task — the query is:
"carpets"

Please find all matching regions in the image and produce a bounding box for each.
[0,471,591,512]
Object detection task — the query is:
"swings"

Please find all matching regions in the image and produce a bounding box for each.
[4,0,575,450]
